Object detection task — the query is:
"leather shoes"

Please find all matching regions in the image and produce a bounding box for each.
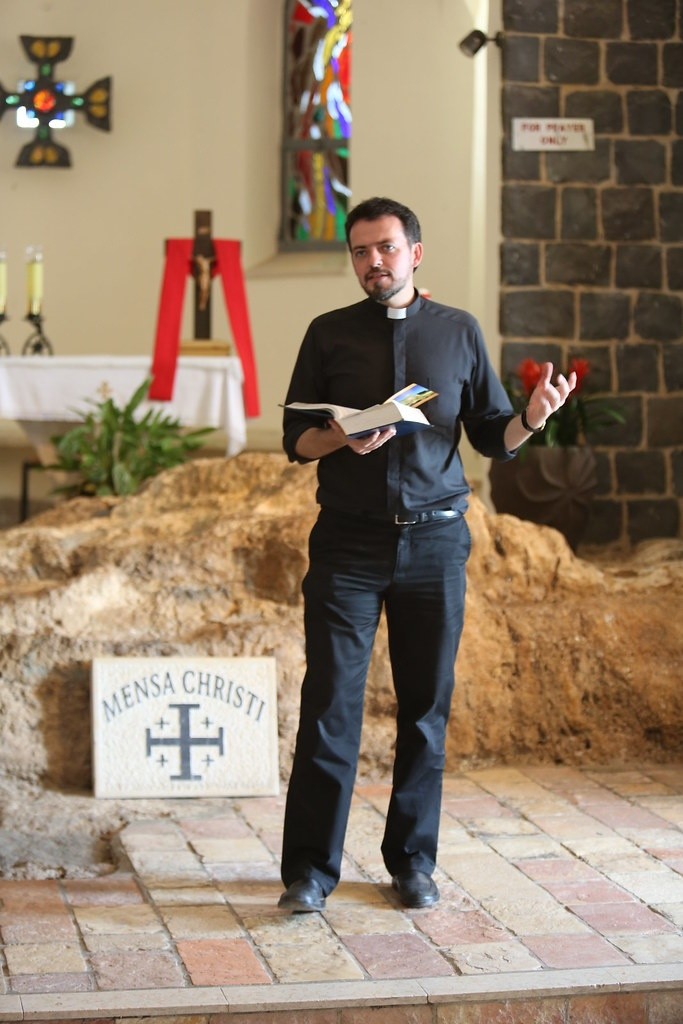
[391,870,440,908]
[278,876,326,911]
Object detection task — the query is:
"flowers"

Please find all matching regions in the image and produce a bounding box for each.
[503,356,629,462]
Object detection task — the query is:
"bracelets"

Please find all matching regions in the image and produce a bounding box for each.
[520,406,546,433]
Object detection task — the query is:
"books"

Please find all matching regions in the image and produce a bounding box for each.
[277,382,439,437]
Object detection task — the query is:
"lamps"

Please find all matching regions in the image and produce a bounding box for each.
[460,30,502,57]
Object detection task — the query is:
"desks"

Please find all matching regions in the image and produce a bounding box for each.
[2,356,248,461]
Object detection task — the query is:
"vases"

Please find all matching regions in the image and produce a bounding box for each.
[486,443,614,561]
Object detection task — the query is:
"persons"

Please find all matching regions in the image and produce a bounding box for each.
[276,197,577,912]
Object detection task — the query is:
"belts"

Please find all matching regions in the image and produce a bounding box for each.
[323,504,461,525]
